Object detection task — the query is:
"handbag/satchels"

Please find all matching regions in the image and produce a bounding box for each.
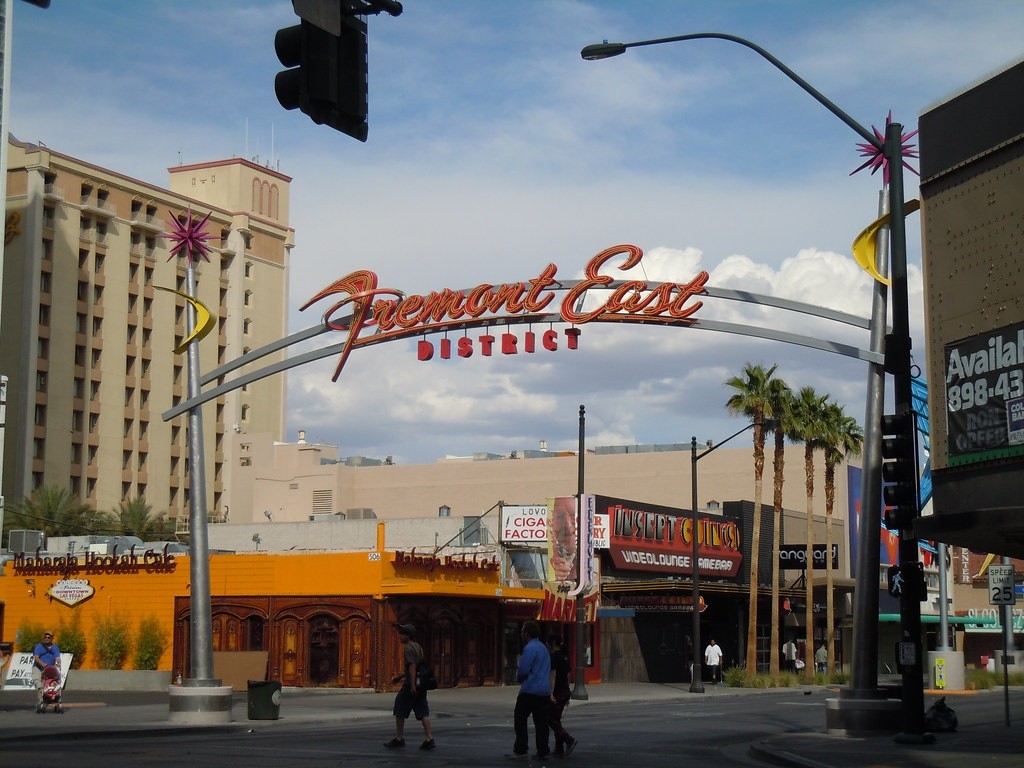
[795,657,804,670]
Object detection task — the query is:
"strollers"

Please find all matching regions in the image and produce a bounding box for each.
[35,664,64,714]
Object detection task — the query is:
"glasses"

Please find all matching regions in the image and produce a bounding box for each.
[44,636,52,640]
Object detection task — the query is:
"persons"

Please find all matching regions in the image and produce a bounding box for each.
[551,498,578,581]
[504,621,552,761]
[705,639,723,685]
[383,623,436,749]
[782,637,798,675]
[816,644,828,675]
[546,632,578,756]
[32,632,61,713]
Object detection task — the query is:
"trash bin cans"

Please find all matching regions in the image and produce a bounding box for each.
[247,680,281,720]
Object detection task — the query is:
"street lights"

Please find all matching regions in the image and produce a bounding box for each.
[580,33,936,746]
[690,418,770,693]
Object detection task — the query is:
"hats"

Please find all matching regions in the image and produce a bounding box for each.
[399,627,414,637]
[45,631,53,637]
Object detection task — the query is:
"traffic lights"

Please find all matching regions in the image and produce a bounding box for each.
[881,414,911,533]
[888,563,904,597]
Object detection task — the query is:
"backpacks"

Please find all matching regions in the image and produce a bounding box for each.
[408,642,438,690]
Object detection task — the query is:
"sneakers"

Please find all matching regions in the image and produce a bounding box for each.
[565,738,579,756]
[505,750,530,761]
[548,751,564,759]
[419,737,437,750]
[531,753,549,762]
[384,738,406,748]
